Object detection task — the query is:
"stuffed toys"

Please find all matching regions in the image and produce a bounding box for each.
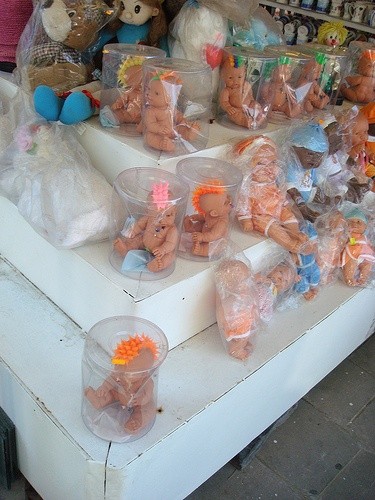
[20,0,345,125]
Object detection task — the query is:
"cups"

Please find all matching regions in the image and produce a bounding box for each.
[260,0,375,45]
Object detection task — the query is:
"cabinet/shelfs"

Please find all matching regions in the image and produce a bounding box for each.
[0,71,375,500]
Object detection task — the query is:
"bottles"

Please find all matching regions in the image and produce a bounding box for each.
[107,166,191,281]
[78,315,169,444]
[176,154,244,261]
[100,43,211,157]
[216,40,375,134]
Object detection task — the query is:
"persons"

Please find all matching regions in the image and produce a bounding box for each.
[85,53,375,433]
